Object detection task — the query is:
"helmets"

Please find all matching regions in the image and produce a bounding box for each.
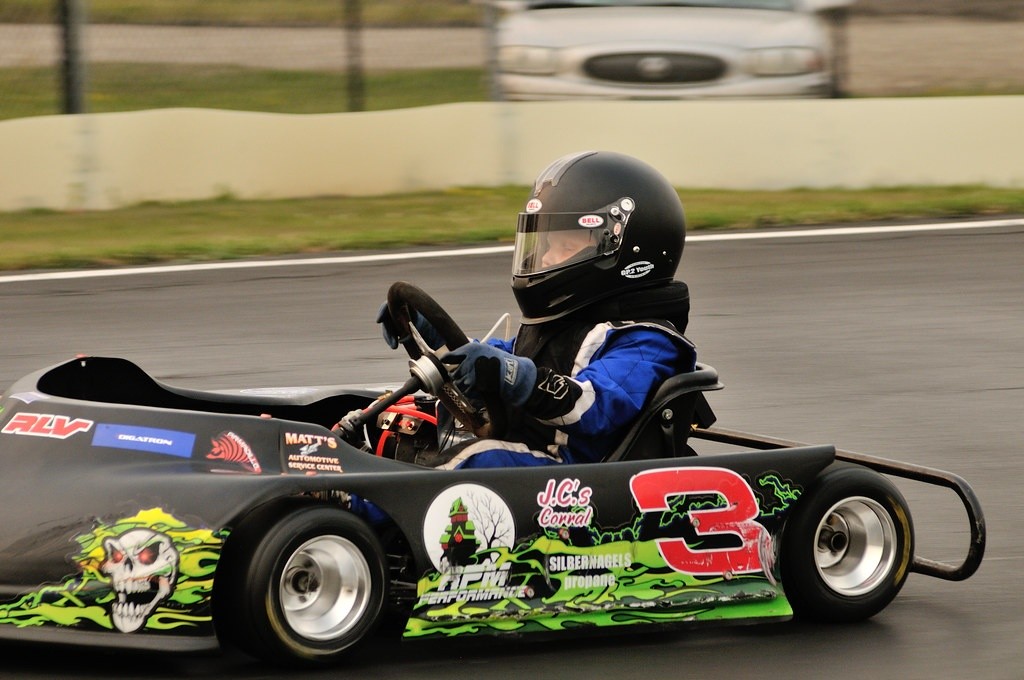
[510,149,687,326]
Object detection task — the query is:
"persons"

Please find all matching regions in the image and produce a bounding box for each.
[377,151,697,471]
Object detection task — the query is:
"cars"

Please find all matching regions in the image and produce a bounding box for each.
[488,1,851,108]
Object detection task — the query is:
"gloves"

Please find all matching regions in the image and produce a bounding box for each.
[377,299,436,358]
[440,341,537,411]
